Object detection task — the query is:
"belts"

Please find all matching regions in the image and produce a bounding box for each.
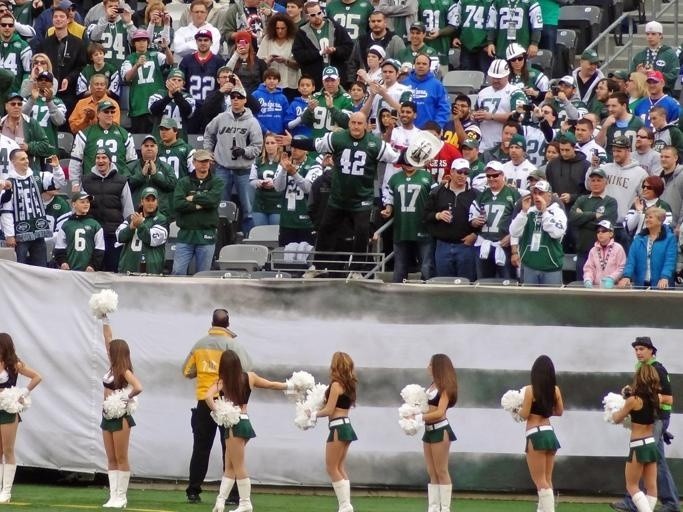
[239,414,249,420]
[630,437,656,448]
[426,419,449,431]
[525,425,553,437]
[330,418,349,427]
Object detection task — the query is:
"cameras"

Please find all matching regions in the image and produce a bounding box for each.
[522,104,534,111]
[44,158,52,164]
[551,85,561,94]
[158,11,166,18]
[114,8,124,13]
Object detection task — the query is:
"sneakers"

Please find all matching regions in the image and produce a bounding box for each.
[346,273,364,280]
[187,494,202,503]
[302,264,329,278]
[655,505,679,512]
[610,502,631,512]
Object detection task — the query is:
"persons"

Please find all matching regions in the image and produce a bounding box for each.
[182,309,250,503]
[315,352,359,512]
[416,352,457,512]
[614,363,662,512]
[608,337,678,512]
[97,313,144,509]
[205,350,288,511]
[0,334,41,504]
[2,0,683,289]
[513,356,564,511]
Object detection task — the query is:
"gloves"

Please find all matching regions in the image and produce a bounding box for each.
[601,277,614,289]
[584,280,593,288]
[231,146,245,157]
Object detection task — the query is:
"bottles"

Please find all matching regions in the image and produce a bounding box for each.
[444,203,453,224]
[477,203,486,226]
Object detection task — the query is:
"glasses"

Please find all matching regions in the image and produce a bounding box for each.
[643,184,651,188]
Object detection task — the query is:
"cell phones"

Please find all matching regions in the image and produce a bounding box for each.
[391,109,397,117]
[370,117,376,127]
[229,75,235,84]
[239,40,246,46]
[451,102,458,113]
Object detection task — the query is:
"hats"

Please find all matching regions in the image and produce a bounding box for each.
[632,336,657,352]
[229,21,426,96]
[488,21,664,84]
[450,130,631,232]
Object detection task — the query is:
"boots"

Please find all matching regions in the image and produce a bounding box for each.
[427,483,440,512]
[439,485,452,512]
[0,463,16,502]
[332,480,353,512]
[229,477,253,512]
[102,469,130,508]
[212,477,235,512]
[631,488,656,512]
[536,488,554,512]
